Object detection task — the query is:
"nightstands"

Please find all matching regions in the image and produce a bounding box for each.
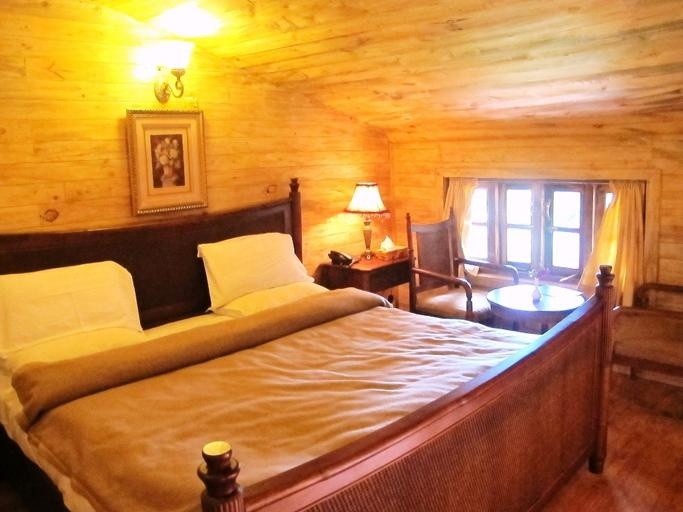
[321,255,410,293]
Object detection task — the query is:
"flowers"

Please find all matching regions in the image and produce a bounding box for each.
[528,263,548,281]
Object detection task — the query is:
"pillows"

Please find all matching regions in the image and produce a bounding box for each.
[195,231,330,316]
[1,260,147,376]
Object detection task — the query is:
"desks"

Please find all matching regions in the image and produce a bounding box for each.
[485,284,586,335]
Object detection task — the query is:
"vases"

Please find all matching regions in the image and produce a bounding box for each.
[531,286,545,301]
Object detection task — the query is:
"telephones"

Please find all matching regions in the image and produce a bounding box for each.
[328,250,353,266]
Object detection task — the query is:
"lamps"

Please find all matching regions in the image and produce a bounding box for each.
[342,182,391,259]
[124,104,210,218]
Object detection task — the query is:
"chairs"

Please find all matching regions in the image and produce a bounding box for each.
[403,204,520,322]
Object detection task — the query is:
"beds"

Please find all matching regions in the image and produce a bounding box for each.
[0,173,618,512]
